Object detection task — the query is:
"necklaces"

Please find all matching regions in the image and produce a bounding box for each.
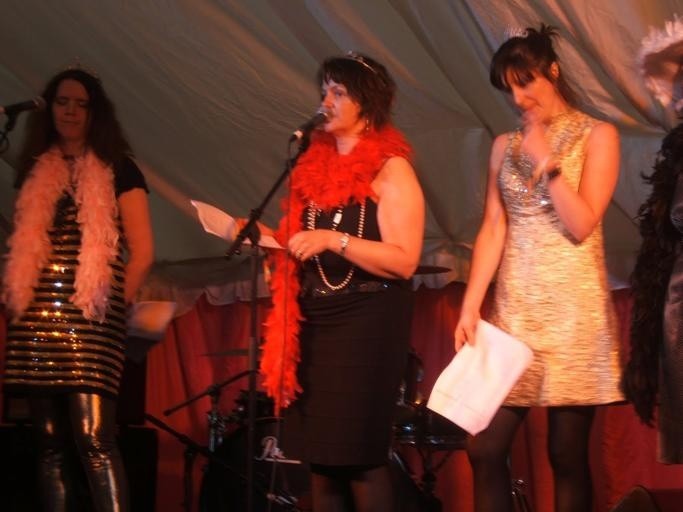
[305,199,366,292]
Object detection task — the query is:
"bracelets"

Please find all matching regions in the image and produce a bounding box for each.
[338,231,350,256]
[533,164,563,184]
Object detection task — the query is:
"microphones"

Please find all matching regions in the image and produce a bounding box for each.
[289,105,333,143]
[0,95,47,114]
[260,421,280,448]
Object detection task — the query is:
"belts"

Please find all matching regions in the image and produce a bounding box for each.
[298,278,393,300]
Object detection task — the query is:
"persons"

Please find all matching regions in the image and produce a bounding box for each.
[130,51,425,511]
[0,66,156,511]
[454,21,630,511]
[617,13,682,467]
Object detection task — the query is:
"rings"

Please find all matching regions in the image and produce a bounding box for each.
[295,249,301,256]
